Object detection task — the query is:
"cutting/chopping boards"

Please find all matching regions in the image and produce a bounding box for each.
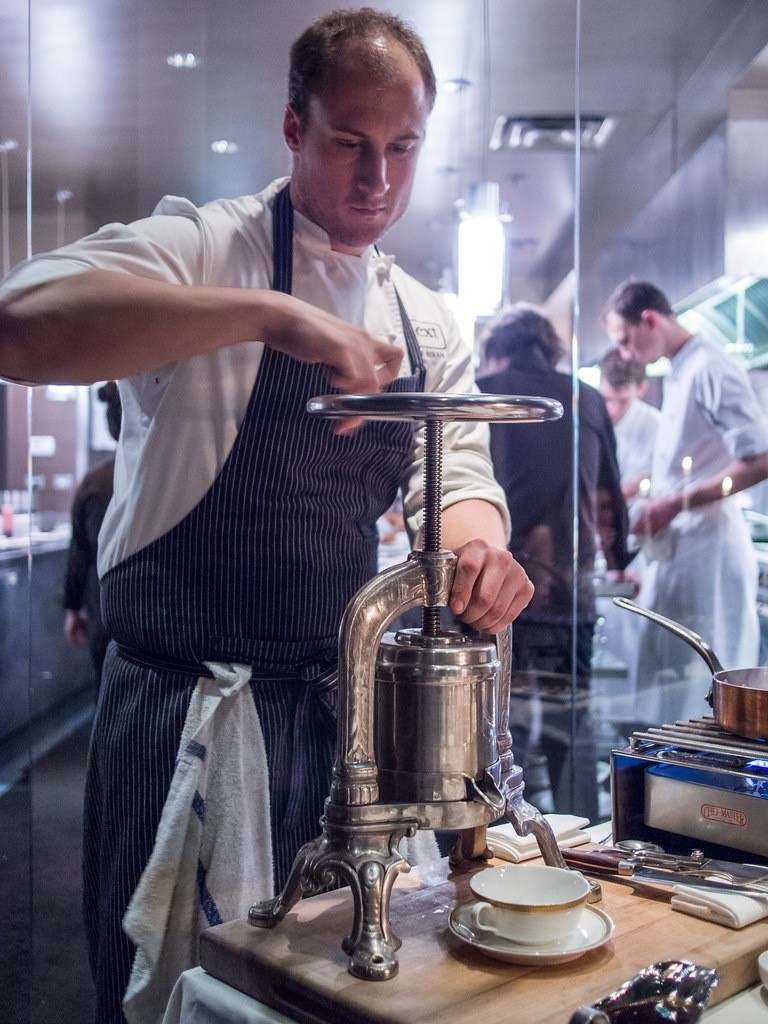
[198,855,768,1024]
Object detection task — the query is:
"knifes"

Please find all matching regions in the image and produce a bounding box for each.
[559,849,768,894]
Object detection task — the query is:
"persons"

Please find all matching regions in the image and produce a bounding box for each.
[470,299,641,828]
[605,277,768,740]
[586,343,665,718]
[58,379,124,718]
[1,8,540,1024]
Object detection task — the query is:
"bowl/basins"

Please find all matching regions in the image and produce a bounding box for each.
[758,951,768,991]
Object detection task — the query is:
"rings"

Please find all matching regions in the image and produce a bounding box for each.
[374,360,386,371]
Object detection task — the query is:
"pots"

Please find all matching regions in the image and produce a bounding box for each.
[613,596,768,738]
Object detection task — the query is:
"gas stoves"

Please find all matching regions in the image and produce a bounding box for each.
[610,715,768,878]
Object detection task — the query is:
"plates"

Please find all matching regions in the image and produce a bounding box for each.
[448,902,616,967]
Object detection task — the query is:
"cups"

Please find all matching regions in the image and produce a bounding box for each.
[469,863,591,947]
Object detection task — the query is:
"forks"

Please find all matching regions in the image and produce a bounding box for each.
[674,865,768,887]
